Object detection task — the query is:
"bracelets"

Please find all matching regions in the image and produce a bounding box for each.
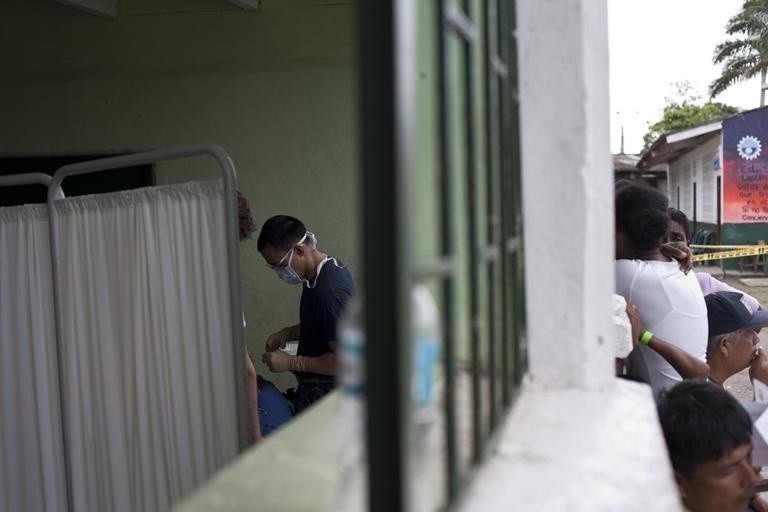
[288,354,307,371]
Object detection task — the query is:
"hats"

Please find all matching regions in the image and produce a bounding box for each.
[705,291,768,335]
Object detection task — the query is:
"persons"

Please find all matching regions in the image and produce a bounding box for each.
[256,216,354,416]
[236,188,264,447]
[613,177,767,510]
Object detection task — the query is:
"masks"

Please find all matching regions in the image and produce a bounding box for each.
[274,247,302,285]
